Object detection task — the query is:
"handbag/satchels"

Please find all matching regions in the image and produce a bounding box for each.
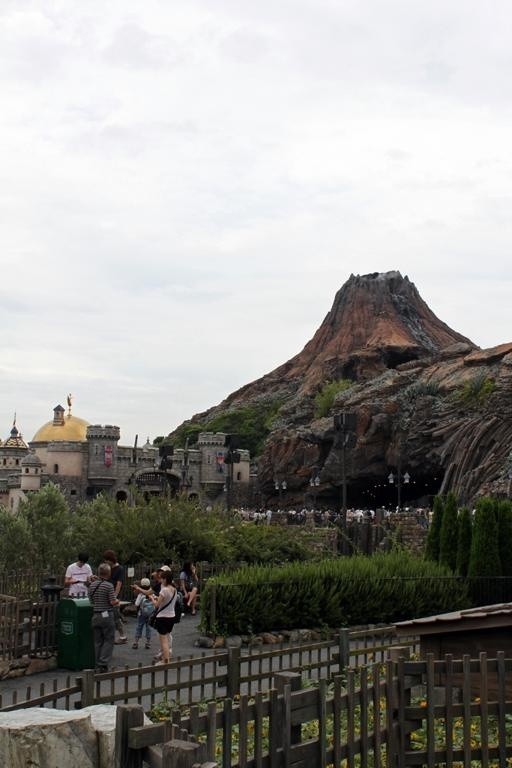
[149,608,157,628]
[174,592,182,623]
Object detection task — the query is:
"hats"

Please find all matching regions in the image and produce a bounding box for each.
[141,577,150,586]
[156,565,171,572]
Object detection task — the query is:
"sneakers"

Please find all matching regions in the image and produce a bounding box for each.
[95,666,108,674]
[115,636,173,666]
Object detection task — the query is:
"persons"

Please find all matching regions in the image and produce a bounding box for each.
[226,506,433,530]
[64,550,203,674]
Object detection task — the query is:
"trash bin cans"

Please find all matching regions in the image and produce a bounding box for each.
[55,597,95,672]
